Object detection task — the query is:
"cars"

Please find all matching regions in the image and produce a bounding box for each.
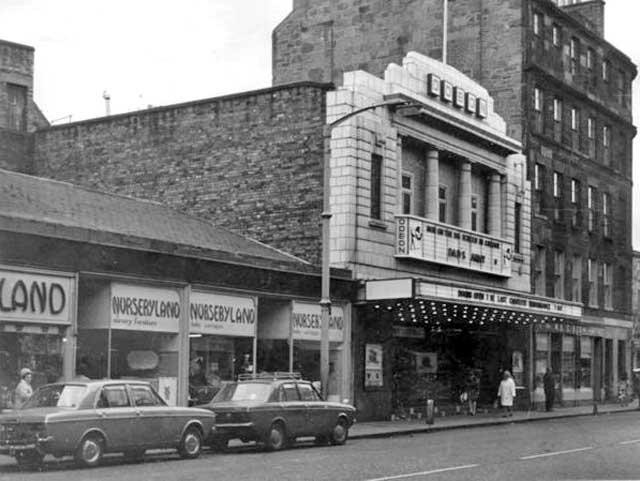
[191,372,357,452]
[0,380,216,467]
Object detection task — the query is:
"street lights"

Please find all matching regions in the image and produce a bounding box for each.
[319,98,424,401]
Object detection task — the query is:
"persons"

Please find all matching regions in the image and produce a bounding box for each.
[534,375,548,411]
[543,366,560,412]
[464,366,479,416]
[619,372,631,407]
[631,367,640,406]
[13,365,35,408]
[389,367,402,421]
[496,368,517,418]
